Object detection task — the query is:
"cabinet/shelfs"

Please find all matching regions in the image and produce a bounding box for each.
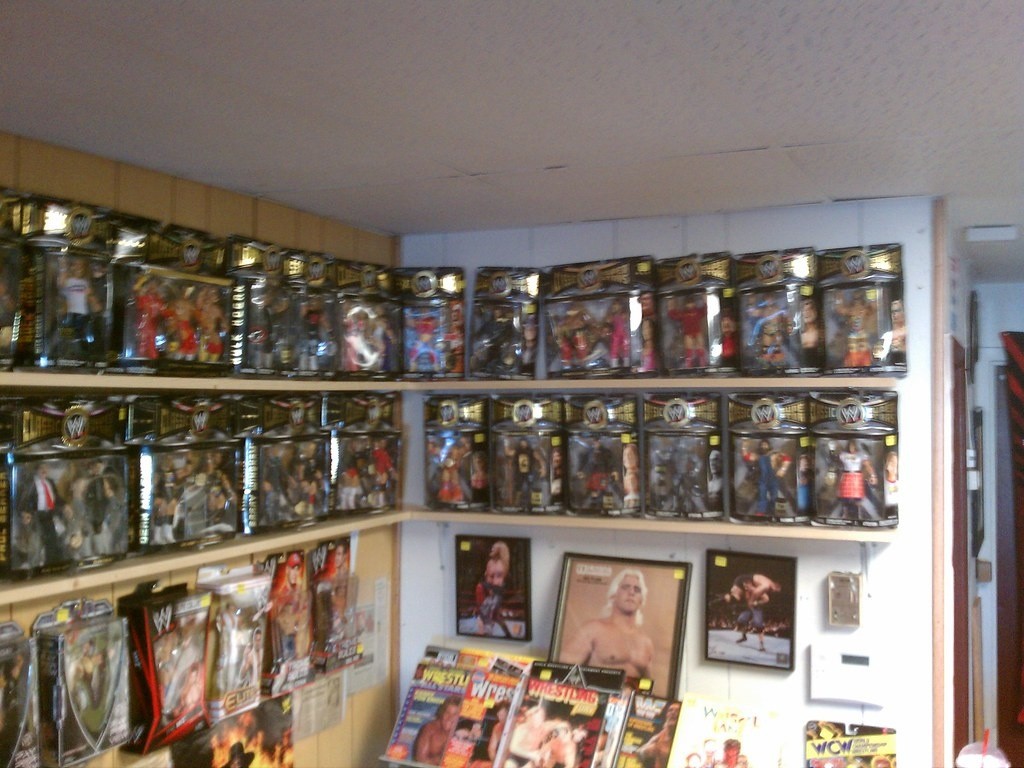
[0,369,900,609]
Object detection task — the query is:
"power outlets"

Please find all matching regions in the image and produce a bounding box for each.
[828,572,862,627]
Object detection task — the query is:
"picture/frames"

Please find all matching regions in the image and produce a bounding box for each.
[972,410,985,558]
[546,551,692,703]
[705,549,799,671]
[455,534,532,642]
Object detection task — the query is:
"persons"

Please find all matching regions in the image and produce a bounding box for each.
[0,586,349,754]
[505,706,589,768]
[888,300,907,351]
[415,699,462,764]
[488,701,511,761]
[686,736,749,768]
[332,542,348,580]
[709,574,789,650]
[884,452,899,506]
[561,568,654,694]
[19,237,881,569]
[473,543,512,636]
[637,701,683,768]
